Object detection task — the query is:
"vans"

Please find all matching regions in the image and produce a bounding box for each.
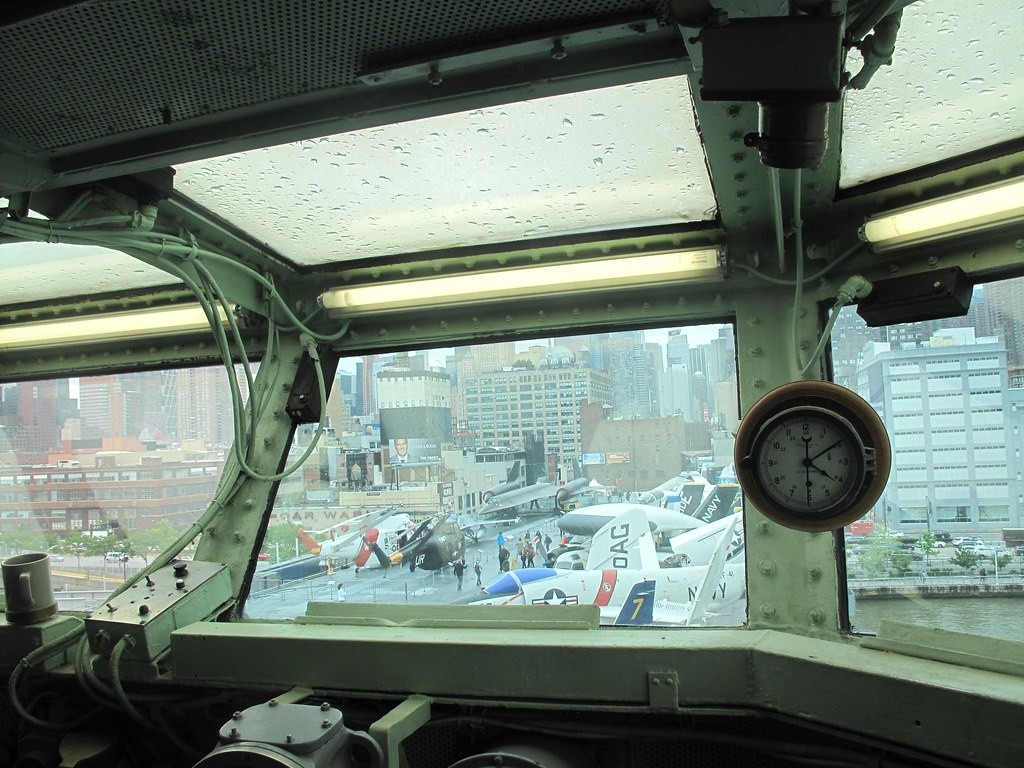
[106,552,129,563]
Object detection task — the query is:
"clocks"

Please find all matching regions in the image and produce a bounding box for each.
[734,380,892,532]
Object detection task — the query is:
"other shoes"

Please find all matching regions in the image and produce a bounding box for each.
[480,581,481,584]
[477,582,479,585]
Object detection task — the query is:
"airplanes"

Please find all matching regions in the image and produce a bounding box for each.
[249,461,745,629]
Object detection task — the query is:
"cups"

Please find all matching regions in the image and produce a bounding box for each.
[1,552,54,612]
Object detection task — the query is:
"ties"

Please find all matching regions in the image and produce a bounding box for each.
[401,460,404,463]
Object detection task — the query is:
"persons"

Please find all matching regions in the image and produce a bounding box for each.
[498,532,505,551]
[515,529,552,569]
[498,545,510,573]
[473,557,483,585]
[454,556,468,591]
[338,583,346,602]
[390,439,420,463]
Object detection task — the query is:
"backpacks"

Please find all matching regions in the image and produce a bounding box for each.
[475,561,480,573]
